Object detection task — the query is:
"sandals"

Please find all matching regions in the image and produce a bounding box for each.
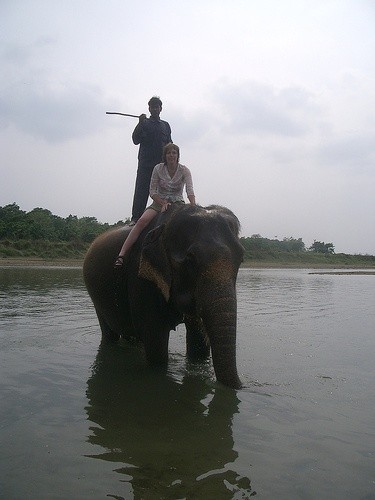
[114,256,125,269]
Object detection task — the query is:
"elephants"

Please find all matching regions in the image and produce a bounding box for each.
[81,200,245,393]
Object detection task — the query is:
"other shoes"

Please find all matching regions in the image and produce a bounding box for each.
[129,221,135,226]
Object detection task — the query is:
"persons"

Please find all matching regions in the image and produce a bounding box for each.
[131,97,174,226]
[114,142,196,269]
